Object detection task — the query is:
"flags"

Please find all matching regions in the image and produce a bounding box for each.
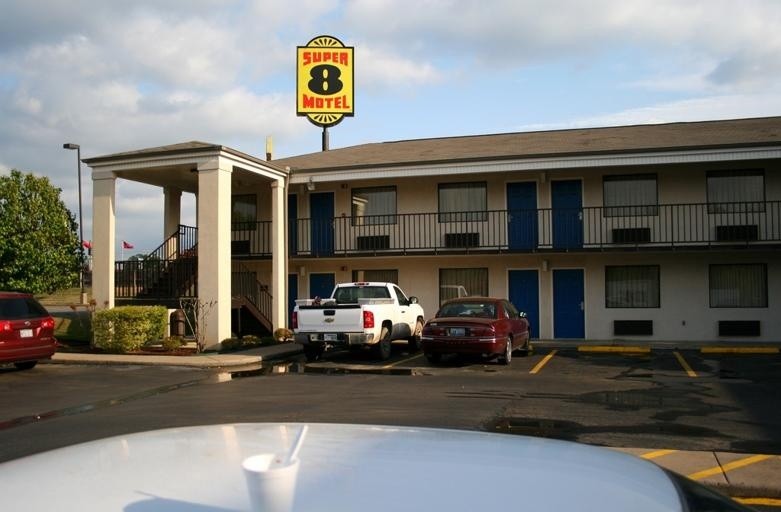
[82,240,91,249]
[124,241,134,248]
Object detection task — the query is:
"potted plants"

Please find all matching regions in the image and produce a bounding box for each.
[275,328,291,343]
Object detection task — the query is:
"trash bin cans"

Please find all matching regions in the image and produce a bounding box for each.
[170,309,186,338]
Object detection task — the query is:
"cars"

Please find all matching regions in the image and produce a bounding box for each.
[421,296,530,365]
[0,291,57,370]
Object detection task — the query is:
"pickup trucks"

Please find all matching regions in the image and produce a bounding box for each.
[292,281,424,362]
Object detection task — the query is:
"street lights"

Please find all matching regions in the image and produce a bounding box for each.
[63,143,85,293]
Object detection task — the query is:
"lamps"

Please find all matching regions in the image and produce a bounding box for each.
[190,168,198,175]
[305,182,315,191]
